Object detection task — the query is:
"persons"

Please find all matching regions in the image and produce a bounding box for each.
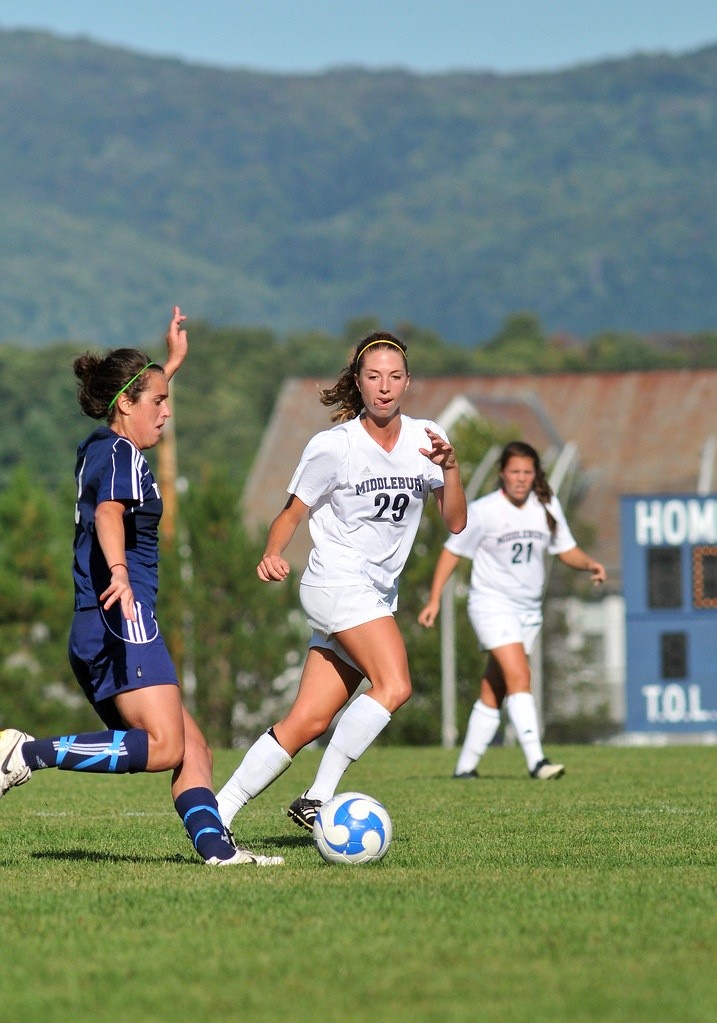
[0,304,290,868]
[418,441,606,782]
[210,330,470,842]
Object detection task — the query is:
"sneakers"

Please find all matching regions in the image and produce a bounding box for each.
[286,789,324,835]
[452,760,565,778]
[0,727,36,795]
[203,849,283,866]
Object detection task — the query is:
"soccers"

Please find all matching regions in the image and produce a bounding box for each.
[313,792,393,867]
